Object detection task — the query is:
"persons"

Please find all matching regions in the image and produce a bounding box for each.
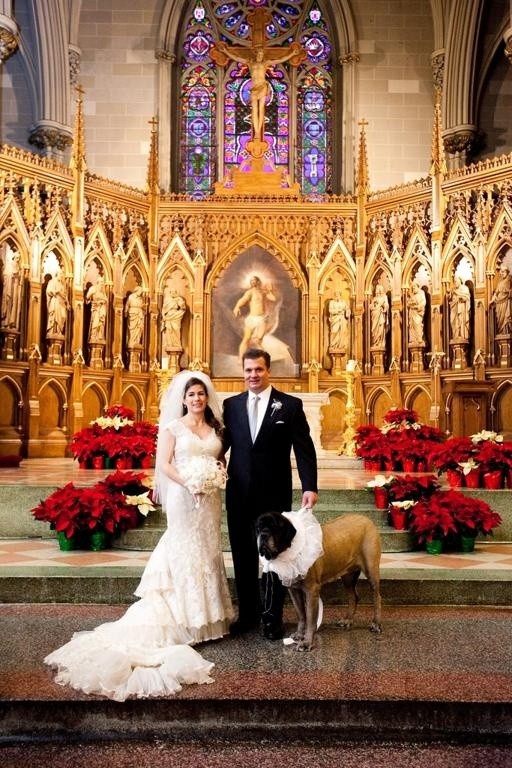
[161,289,186,348]
[215,39,308,140]
[124,285,146,348]
[449,277,470,340]
[489,265,511,334]
[46,269,71,335]
[85,277,108,343]
[1,251,22,328]
[41,369,234,704]
[370,284,389,350]
[329,289,351,350]
[215,348,321,643]
[407,282,426,344]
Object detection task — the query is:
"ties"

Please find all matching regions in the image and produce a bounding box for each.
[249,395,260,445]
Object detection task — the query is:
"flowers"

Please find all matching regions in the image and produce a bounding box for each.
[270,397,283,418]
[351,407,512,543]
[29,403,160,538]
[176,453,229,509]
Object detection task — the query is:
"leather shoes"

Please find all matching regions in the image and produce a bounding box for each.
[265,620,283,638]
[230,620,257,635]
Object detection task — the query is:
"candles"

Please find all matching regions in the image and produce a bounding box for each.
[346,356,356,373]
[161,355,169,372]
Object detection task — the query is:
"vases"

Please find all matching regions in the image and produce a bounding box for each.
[92,531,104,551]
[426,540,443,557]
[459,535,476,552]
[56,530,76,551]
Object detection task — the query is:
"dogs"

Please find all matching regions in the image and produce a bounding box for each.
[254,511,383,653]
